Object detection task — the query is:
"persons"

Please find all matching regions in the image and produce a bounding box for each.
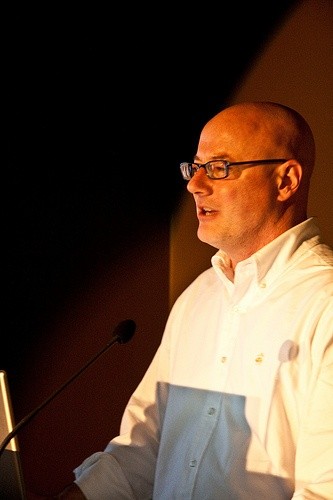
[57,100,333,500]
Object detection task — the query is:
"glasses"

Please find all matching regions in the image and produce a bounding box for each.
[179,159,286,180]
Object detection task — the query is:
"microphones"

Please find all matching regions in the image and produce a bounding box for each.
[0,318,137,456]
